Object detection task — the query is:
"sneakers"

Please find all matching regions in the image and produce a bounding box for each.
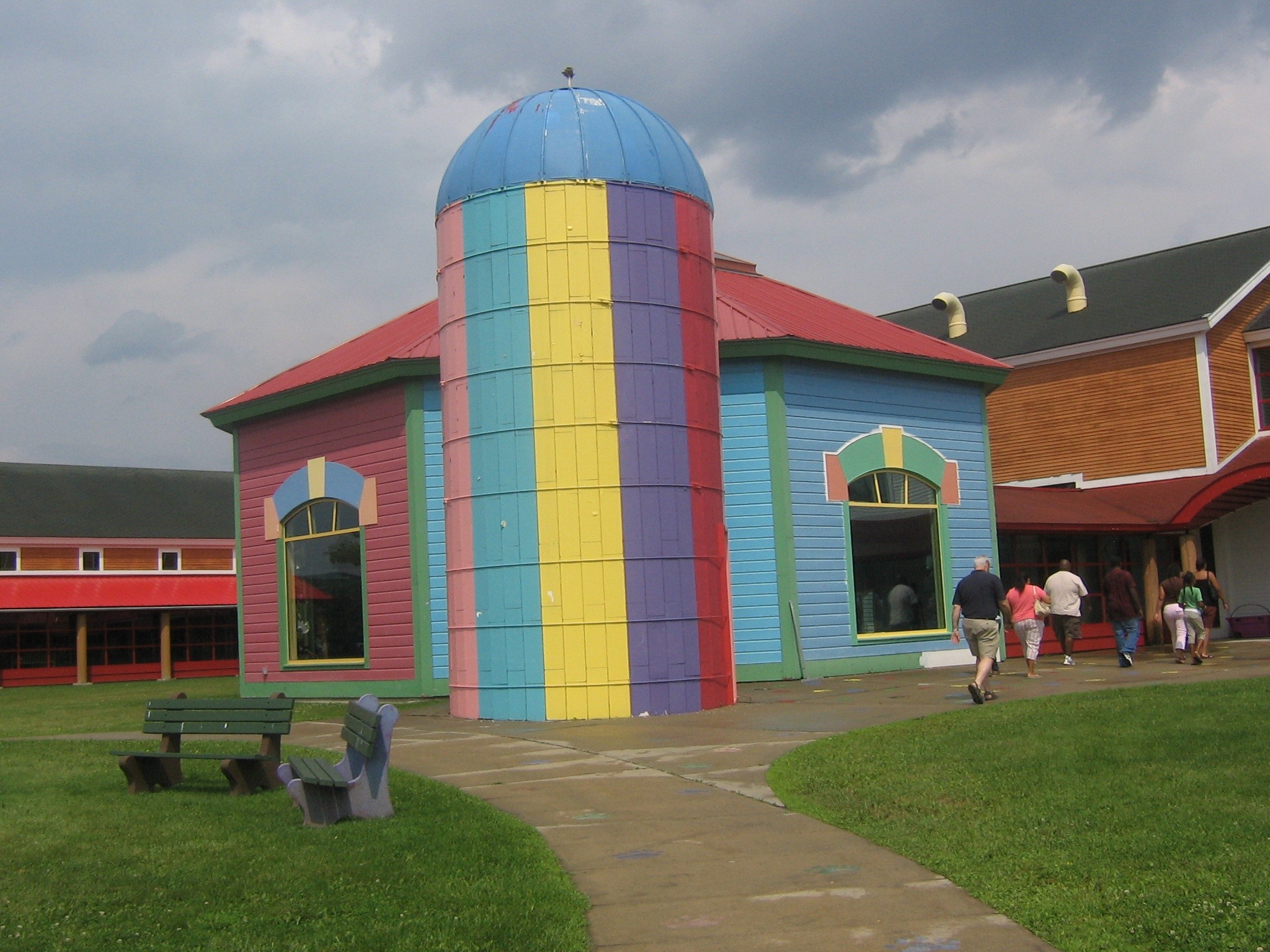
[1063,654,1075,665]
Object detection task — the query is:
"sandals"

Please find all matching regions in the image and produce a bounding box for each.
[968,681,984,704]
[985,690,998,701]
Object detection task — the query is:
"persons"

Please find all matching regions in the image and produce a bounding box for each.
[1044,560,1090,665]
[951,555,1011,704]
[1178,571,1206,665]
[1102,556,1143,667]
[988,610,1002,677]
[1155,563,1190,663]
[1006,573,1050,678]
[1191,557,1228,657]
[856,575,937,631]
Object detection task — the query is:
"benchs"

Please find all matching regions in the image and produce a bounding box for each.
[107,693,295,797]
[276,694,400,827]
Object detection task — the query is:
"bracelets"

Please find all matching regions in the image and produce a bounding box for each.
[951,629,959,632]
[1224,603,1227,604]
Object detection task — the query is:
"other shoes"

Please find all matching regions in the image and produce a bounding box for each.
[1191,661,1197,666]
[1118,651,1133,667]
[1028,673,1041,679]
[1193,651,1203,663]
[1175,655,1186,664]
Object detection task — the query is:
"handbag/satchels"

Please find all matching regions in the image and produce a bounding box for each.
[1033,585,1051,618]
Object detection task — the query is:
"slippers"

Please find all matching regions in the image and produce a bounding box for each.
[1201,653,1213,658]
